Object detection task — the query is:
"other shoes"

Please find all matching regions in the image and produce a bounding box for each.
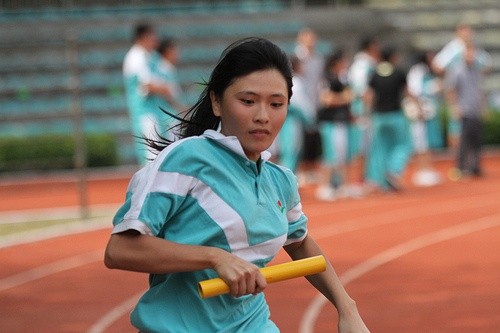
[317,184,363,200]
[413,167,443,184]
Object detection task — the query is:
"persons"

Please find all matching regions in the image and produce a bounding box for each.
[103,37,370,333]
[122,19,489,201]
